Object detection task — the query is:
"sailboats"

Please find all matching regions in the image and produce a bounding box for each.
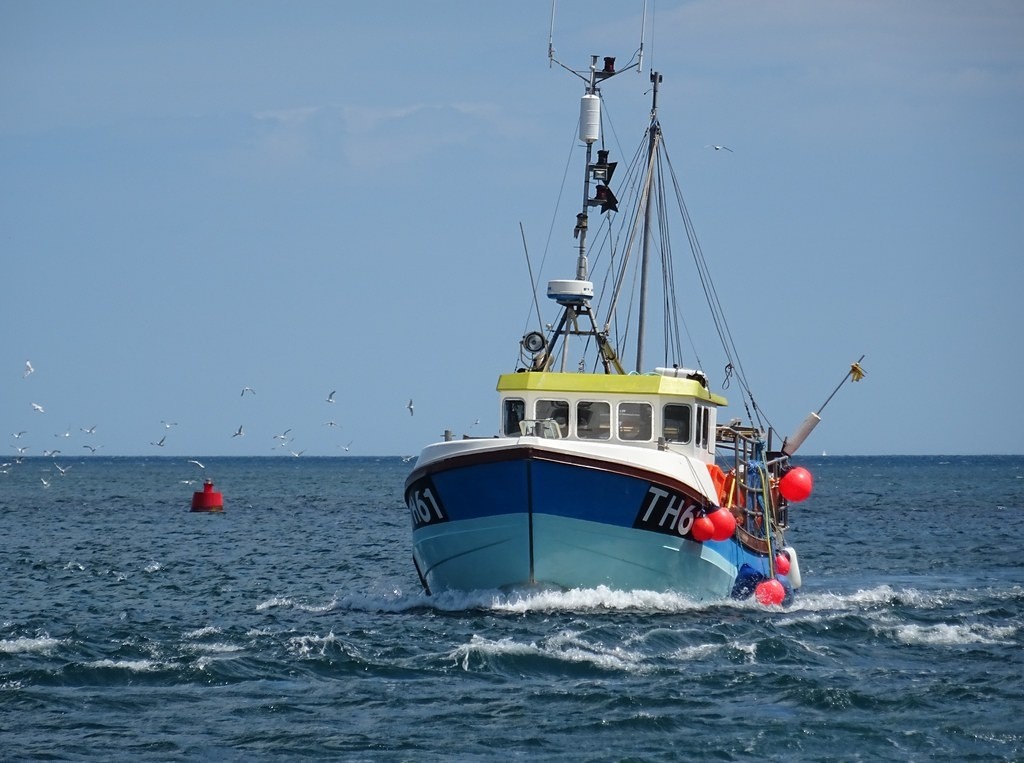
[403,0,866,610]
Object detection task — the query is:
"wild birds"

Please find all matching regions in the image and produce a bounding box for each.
[187,459,206,470]
[9,444,32,465]
[320,420,343,429]
[37,449,74,490]
[21,359,37,381]
[398,454,416,463]
[180,478,199,486]
[231,425,245,438]
[27,400,45,415]
[469,416,481,430]
[240,384,256,398]
[405,397,414,418]
[10,430,28,440]
[324,391,338,406]
[82,445,105,453]
[78,425,99,435]
[0,462,13,475]
[52,428,75,442]
[149,435,166,448]
[160,419,179,430]
[335,440,353,452]
[271,428,309,459]
